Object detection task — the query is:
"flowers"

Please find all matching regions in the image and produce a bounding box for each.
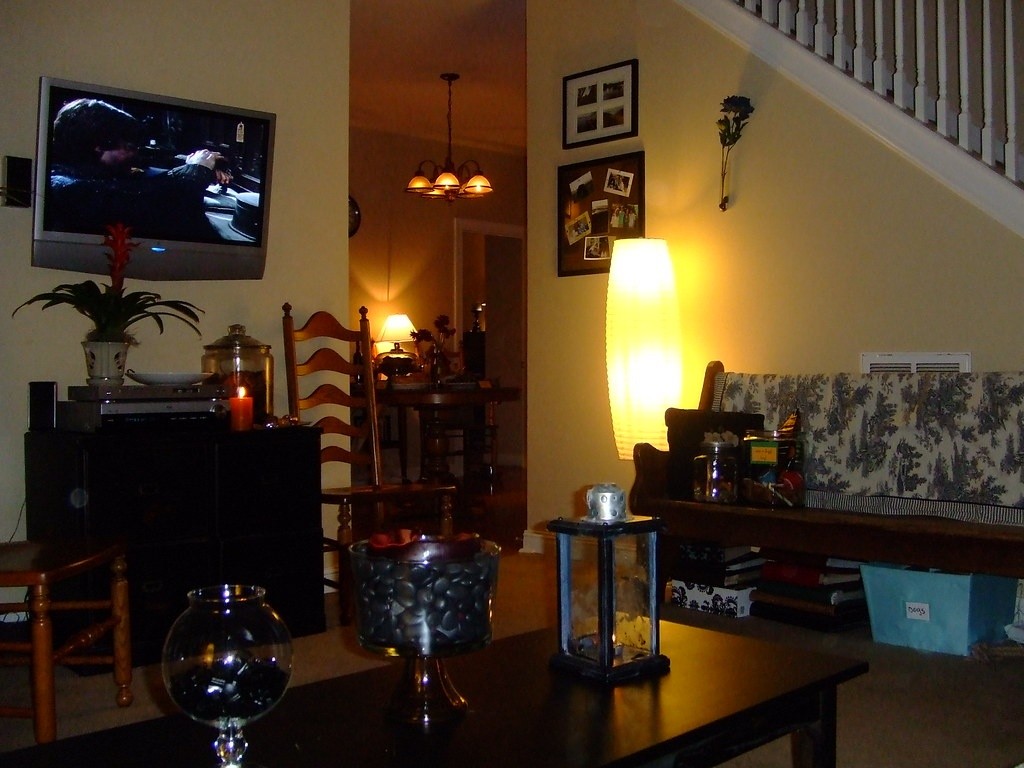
[407,316,453,358]
[12,221,204,340]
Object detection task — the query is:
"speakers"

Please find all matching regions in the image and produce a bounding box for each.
[27,381,57,430]
[2,156,32,208]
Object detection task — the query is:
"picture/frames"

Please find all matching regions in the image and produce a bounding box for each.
[557,153,650,277]
[559,60,638,149]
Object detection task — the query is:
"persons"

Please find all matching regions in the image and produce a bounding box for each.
[610,205,636,227]
[591,239,606,257]
[574,221,583,231]
[50,98,230,238]
[610,176,624,191]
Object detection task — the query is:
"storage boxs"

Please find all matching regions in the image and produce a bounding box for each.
[857,558,1016,659]
[660,579,758,617]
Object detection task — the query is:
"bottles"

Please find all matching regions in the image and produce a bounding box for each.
[692,442,736,503]
[353,341,363,383]
[201,324,274,430]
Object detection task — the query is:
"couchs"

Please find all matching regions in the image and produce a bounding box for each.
[632,360,1022,630]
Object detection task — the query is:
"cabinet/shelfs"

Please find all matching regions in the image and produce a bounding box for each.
[24,416,324,680]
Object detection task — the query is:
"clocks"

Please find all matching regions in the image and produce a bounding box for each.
[347,196,362,239]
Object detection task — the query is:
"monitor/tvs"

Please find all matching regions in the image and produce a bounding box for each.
[30,76,277,281]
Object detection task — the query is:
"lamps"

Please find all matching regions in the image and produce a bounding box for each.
[408,73,497,202]
[228,387,257,430]
[377,313,418,365]
[604,238,682,460]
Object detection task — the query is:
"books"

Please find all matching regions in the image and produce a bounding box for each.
[750,561,863,633]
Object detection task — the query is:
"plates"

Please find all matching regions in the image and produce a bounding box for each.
[124,372,215,387]
[390,383,429,389]
[445,383,480,387]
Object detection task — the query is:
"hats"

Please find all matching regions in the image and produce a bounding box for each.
[54,97,142,151]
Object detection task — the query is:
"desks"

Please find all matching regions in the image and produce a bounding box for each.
[358,383,522,502]
[8,612,872,767]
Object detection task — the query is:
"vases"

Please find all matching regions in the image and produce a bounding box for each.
[427,352,447,380]
[81,338,132,388]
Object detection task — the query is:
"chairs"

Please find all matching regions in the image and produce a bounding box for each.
[281,304,454,620]
[0,539,141,745]
